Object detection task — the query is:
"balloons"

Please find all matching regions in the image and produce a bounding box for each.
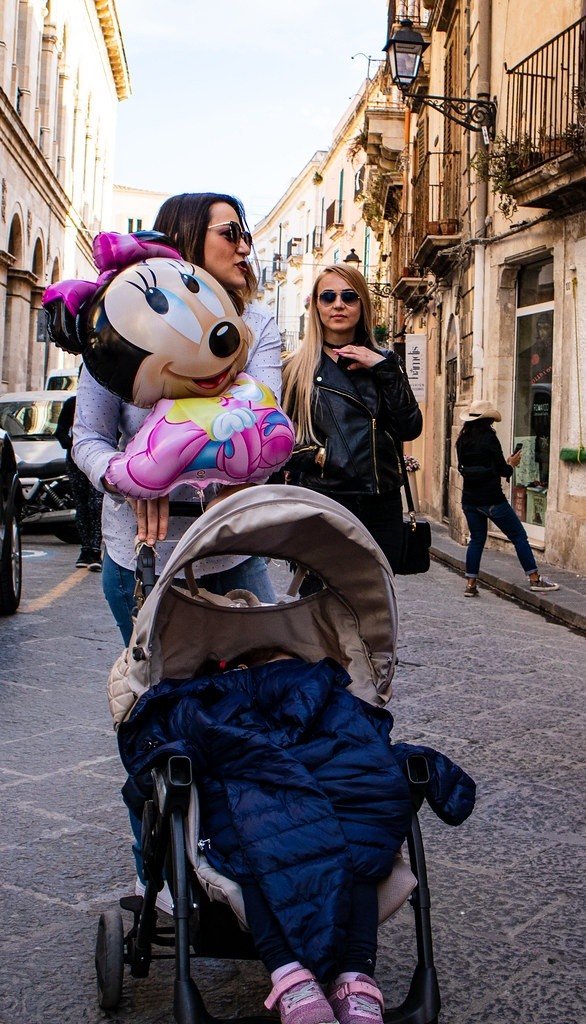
[40,233,296,499]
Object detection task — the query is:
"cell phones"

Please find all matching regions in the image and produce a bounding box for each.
[512,443,523,456]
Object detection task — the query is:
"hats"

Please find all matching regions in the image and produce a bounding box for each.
[458,401,502,423]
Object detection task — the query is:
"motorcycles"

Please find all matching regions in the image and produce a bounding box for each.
[15,458,82,544]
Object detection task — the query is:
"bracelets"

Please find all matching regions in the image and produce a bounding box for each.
[316,446,325,465]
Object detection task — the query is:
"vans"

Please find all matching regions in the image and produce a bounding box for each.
[44,367,80,392]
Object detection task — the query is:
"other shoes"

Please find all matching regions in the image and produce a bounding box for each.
[529,574,560,591]
[134,875,196,916]
[464,585,479,597]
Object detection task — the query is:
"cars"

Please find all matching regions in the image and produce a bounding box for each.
[532,381,551,439]
[0,428,24,617]
[0,391,76,463]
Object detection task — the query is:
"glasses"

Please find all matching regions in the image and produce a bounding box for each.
[208,220,252,249]
[317,291,361,306]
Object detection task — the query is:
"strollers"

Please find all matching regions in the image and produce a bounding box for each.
[94,481,443,1024]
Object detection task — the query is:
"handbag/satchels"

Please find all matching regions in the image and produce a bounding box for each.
[395,520,431,575]
[107,648,139,733]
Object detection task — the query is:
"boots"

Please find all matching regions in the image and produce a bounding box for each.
[75,546,103,571]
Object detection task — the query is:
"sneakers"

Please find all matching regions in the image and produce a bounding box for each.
[328,971,384,1024]
[264,965,340,1024]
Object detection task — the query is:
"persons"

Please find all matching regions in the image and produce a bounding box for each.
[190,645,388,1023]
[72,192,281,915]
[455,401,560,597]
[280,266,424,599]
[54,362,103,571]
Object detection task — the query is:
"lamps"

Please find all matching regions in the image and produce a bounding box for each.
[344,249,391,297]
[384,14,496,141]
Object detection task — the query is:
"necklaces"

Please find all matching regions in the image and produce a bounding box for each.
[325,340,356,350]
